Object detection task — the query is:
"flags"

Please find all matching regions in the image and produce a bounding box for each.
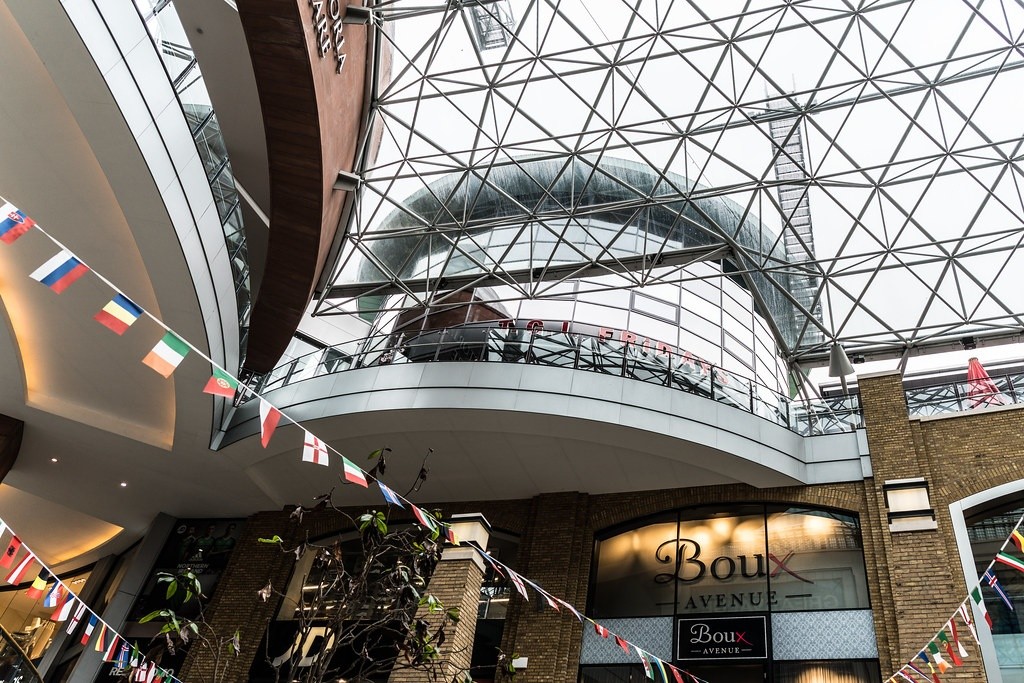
[476,548,699,683]
[890,530,1024,683]
[93,293,144,336]
[377,483,403,508]
[259,400,282,448]
[0,202,35,244]
[440,527,460,546]
[203,369,238,399]
[0,526,172,683]
[141,331,191,379]
[29,250,89,296]
[302,430,329,467]
[343,457,368,488]
[412,505,436,532]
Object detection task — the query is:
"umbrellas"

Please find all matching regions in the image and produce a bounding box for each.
[967,358,1006,410]
